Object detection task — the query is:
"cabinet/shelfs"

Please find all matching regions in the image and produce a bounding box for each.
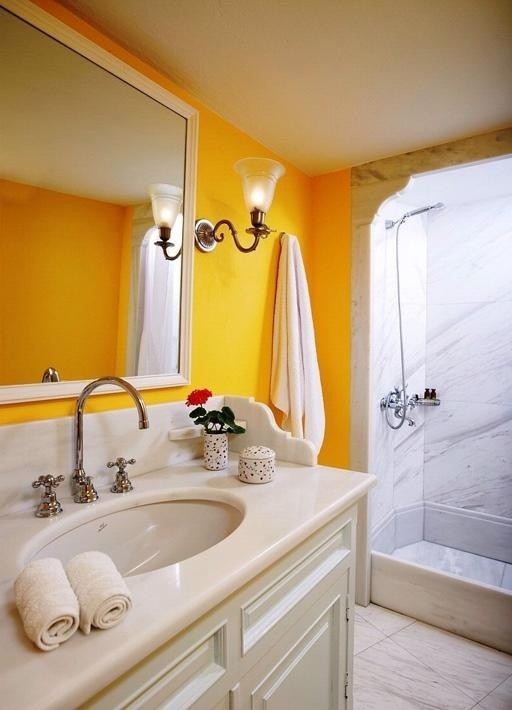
[80,498,361,710]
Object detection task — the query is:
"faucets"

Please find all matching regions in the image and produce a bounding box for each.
[40,367,61,382]
[71,375,148,504]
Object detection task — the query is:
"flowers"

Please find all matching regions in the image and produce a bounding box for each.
[186,385,248,438]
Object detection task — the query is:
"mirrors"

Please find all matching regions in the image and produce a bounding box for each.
[0,0,200,408]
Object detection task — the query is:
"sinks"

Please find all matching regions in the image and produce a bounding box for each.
[20,486,246,579]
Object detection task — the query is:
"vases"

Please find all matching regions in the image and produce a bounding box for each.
[202,427,230,471]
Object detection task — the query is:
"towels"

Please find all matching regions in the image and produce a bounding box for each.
[268,226,327,455]
[11,546,138,653]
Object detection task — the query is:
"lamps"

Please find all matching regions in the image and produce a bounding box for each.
[150,182,184,264]
[196,155,287,256]
